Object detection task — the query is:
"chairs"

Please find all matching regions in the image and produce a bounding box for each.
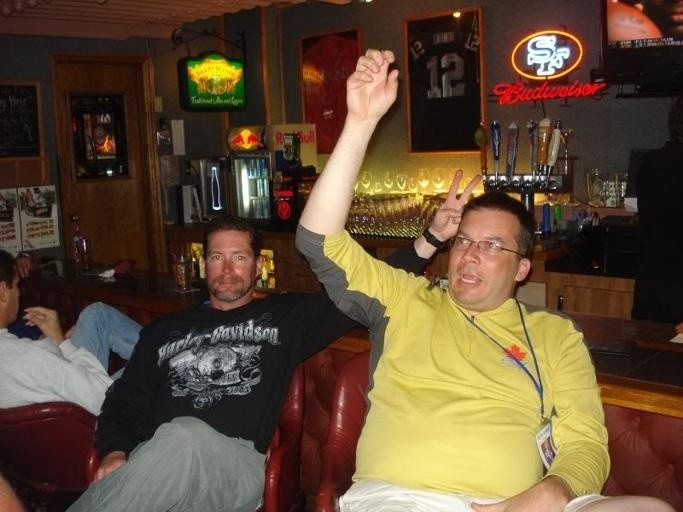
[315,351,378,512]
[0,401,98,512]
[260,360,308,512]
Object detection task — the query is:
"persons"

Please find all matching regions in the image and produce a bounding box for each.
[674,321,682,334]
[61,167,486,512]
[12,250,40,281]
[158,120,170,137]
[293,48,680,512]
[633,96,682,323]
[0,473,25,511]
[0,249,145,420]
[19,187,47,210]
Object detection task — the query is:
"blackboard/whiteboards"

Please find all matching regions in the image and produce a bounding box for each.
[0,80,46,162]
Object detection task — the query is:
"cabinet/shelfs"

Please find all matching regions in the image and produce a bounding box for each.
[274,232,380,334]
[551,270,636,319]
[439,246,559,319]
[380,241,439,288]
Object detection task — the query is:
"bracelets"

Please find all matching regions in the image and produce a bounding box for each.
[420,225,447,252]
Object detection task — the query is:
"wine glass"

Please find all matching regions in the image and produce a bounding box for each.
[352,167,464,196]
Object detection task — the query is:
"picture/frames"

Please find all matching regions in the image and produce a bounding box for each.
[295,21,367,159]
[64,86,132,184]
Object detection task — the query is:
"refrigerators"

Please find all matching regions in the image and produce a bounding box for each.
[225,122,273,222]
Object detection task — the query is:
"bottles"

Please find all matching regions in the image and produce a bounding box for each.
[192,246,274,291]
[585,166,627,210]
[542,203,551,232]
[70,214,90,259]
[172,252,194,293]
[520,180,535,213]
[553,204,562,226]
[210,167,222,211]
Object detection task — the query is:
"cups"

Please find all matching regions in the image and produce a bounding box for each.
[348,198,433,234]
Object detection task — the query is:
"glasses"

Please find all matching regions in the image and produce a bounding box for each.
[449,235,529,260]
